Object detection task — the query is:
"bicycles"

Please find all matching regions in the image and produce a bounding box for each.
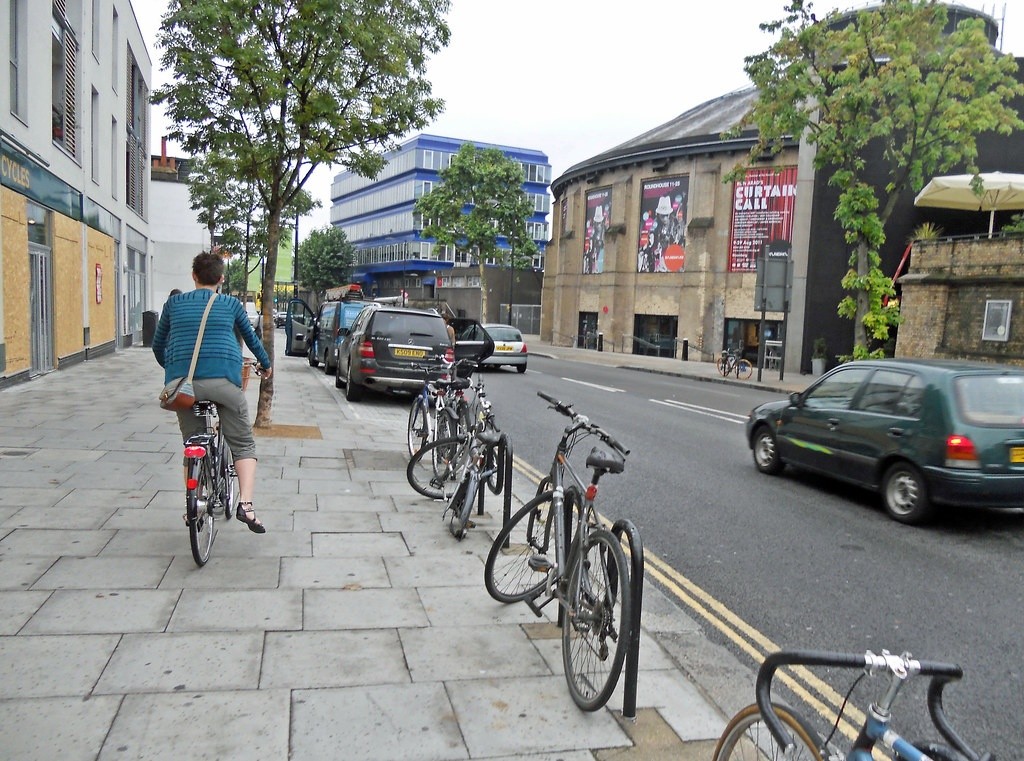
[182,359,265,566]
[484,391,630,712]
[717,350,754,380]
[712,649,992,761]
[407,355,502,540]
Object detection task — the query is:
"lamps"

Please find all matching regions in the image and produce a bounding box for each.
[653,164,667,172]
[756,155,774,162]
[586,176,598,183]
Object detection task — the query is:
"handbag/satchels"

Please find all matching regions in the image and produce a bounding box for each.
[159,376,194,410]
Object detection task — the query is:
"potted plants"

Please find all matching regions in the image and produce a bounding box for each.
[810,337,828,375]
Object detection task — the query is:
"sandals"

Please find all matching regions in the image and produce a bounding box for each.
[182,511,204,532]
[236,502,265,533]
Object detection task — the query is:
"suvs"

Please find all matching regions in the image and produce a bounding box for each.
[239,302,261,340]
[335,306,495,408]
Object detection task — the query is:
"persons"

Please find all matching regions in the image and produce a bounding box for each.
[152,253,272,534]
[641,195,684,273]
[441,313,456,350]
[586,205,607,274]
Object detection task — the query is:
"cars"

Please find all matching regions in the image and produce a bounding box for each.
[285,298,383,375]
[457,323,528,373]
[273,309,306,328]
[745,359,1024,525]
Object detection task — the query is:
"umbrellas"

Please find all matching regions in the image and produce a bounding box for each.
[914,170,1024,237]
